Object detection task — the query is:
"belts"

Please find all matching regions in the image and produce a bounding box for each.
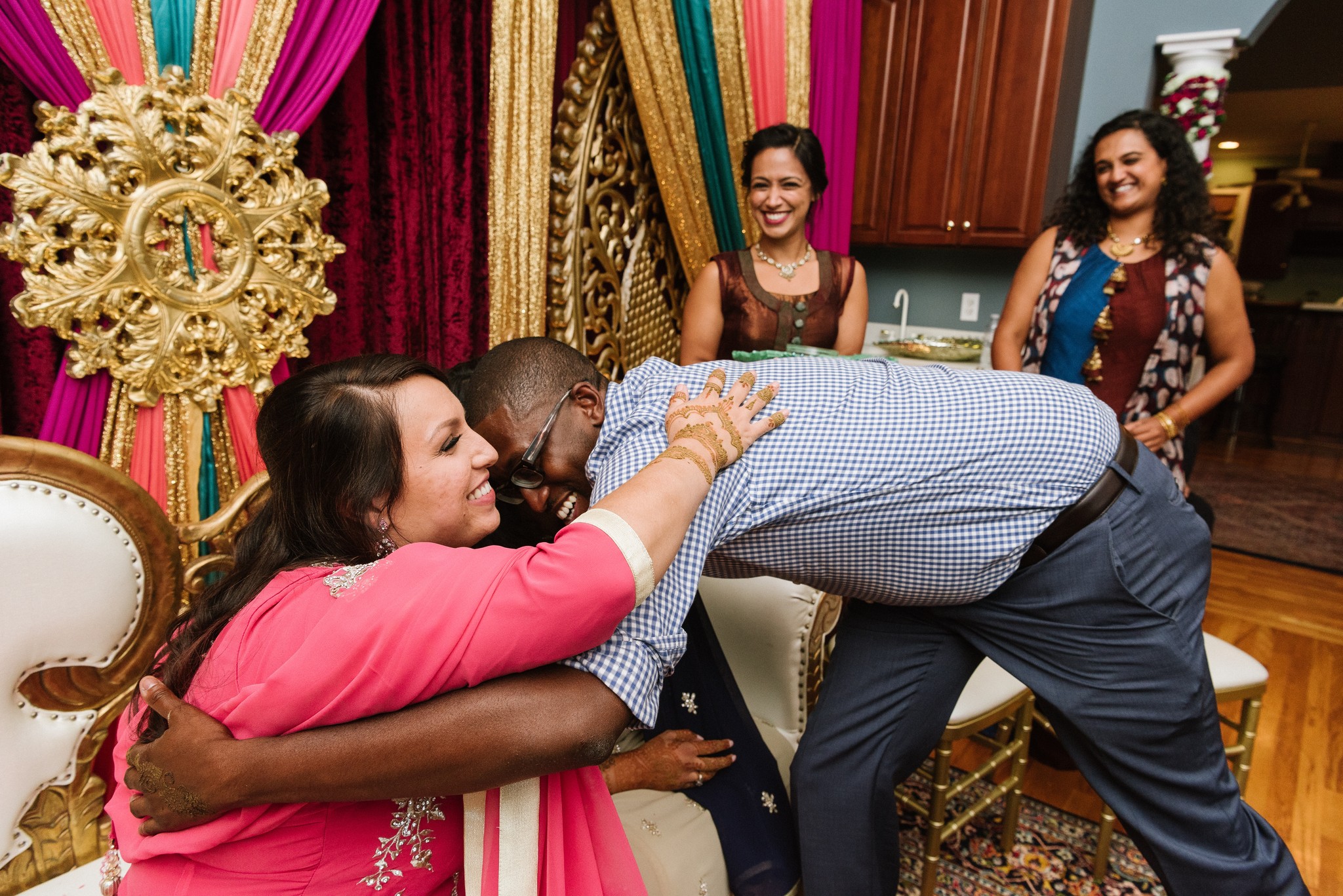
[992,399,1140,589]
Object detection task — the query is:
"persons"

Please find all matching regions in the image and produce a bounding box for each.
[991,111,1256,498]
[106,355,792,896]
[125,337,1311,896]
[684,124,868,367]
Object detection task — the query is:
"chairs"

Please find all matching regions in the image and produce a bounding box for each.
[1089,630,1268,881]
[892,657,1033,893]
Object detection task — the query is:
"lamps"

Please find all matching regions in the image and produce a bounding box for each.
[1217,117,1334,178]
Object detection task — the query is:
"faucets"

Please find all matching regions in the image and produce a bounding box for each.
[893,289,910,340]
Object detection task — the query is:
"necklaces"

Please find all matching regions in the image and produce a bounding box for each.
[755,241,812,281]
[1107,221,1152,257]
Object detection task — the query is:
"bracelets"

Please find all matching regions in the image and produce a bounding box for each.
[1154,411,1178,440]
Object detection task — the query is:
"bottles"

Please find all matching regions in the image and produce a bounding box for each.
[978,314,999,372]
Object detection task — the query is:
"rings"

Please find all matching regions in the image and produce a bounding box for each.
[696,772,703,786]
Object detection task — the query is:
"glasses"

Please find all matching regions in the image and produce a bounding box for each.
[487,388,579,508]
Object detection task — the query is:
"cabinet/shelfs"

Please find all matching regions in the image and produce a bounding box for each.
[844,1,1094,260]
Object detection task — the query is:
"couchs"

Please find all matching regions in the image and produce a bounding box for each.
[2,430,839,896]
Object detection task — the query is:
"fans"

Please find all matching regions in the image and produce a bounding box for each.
[1272,174,1329,212]
